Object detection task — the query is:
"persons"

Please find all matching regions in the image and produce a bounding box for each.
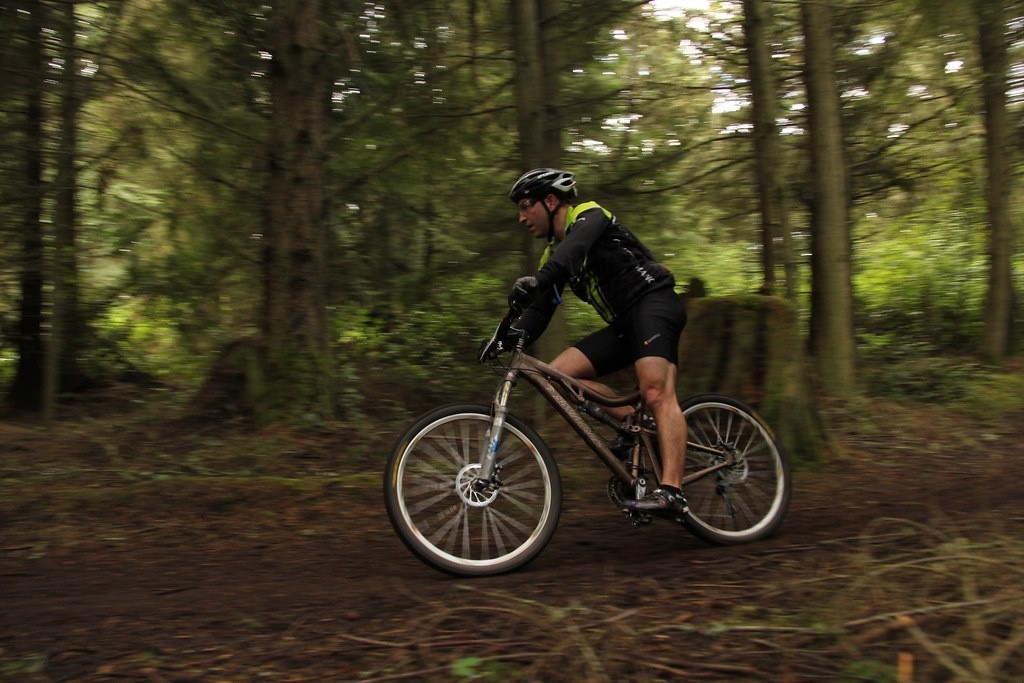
[477,167,688,517]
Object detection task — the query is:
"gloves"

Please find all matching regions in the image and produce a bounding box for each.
[512,276,538,301]
[477,338,505,364]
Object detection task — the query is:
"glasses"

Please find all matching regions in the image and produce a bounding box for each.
[520,199,535,212]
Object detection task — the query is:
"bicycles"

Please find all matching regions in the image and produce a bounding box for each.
[384,283,789,578]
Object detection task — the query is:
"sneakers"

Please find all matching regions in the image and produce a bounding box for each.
[621,489,689,517]
[610,413,656,457]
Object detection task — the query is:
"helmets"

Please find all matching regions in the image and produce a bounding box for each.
[508,168,576,203]
